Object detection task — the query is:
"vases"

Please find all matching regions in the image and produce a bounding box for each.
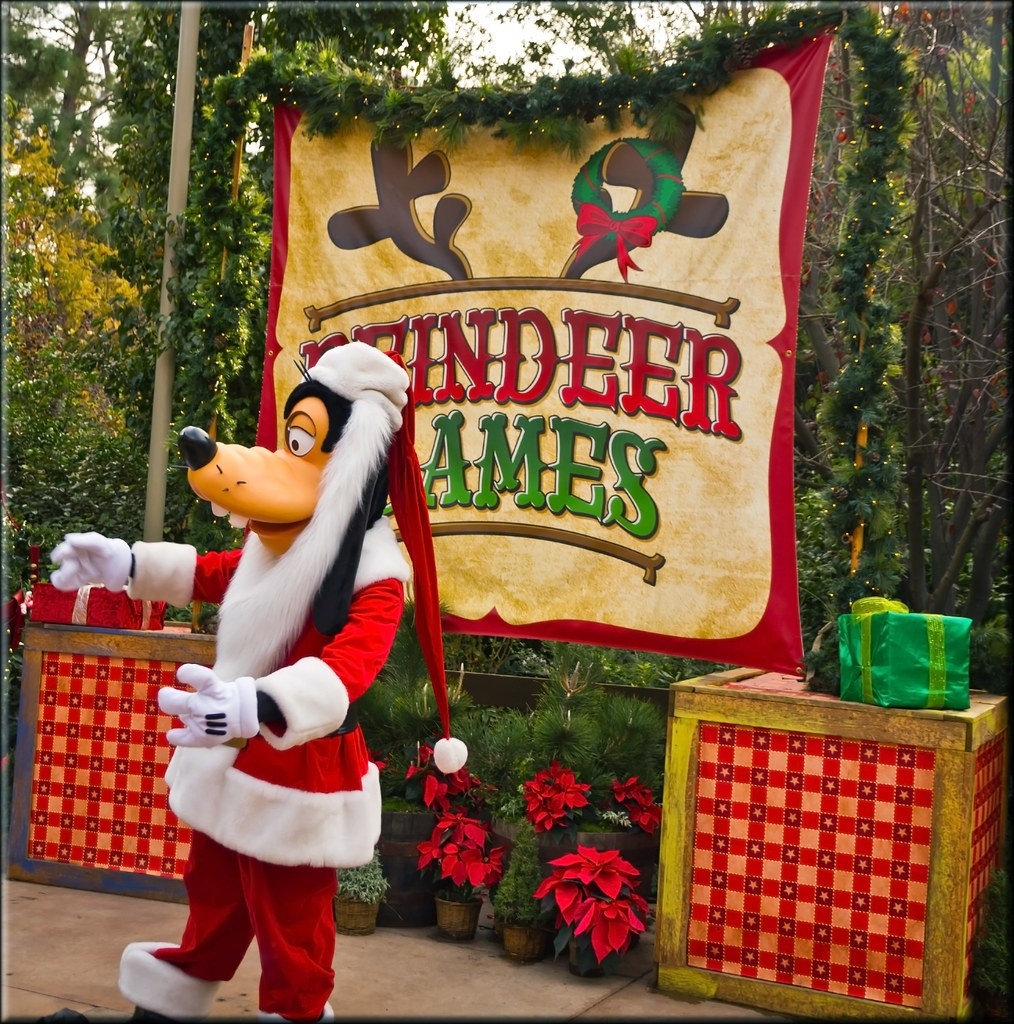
[565,932,617,980]
[434,889,483,944]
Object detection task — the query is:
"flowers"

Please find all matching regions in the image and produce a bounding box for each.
[598,776,664,836]
[406,739,498,810]
[412,807,510,902]
[532,844,657,967]
[522,759,593,834]
[366,746,389,770]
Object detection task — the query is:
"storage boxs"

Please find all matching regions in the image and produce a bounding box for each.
[651,663,1010,1024]
[5,625,219,907]
[836,612,972,711]
[28,580,165,630]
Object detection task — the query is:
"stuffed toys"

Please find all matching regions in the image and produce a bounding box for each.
[42,339,468,1024]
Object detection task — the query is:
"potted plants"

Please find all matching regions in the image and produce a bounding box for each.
[330,847,392,937]
[491,815,551,962]
